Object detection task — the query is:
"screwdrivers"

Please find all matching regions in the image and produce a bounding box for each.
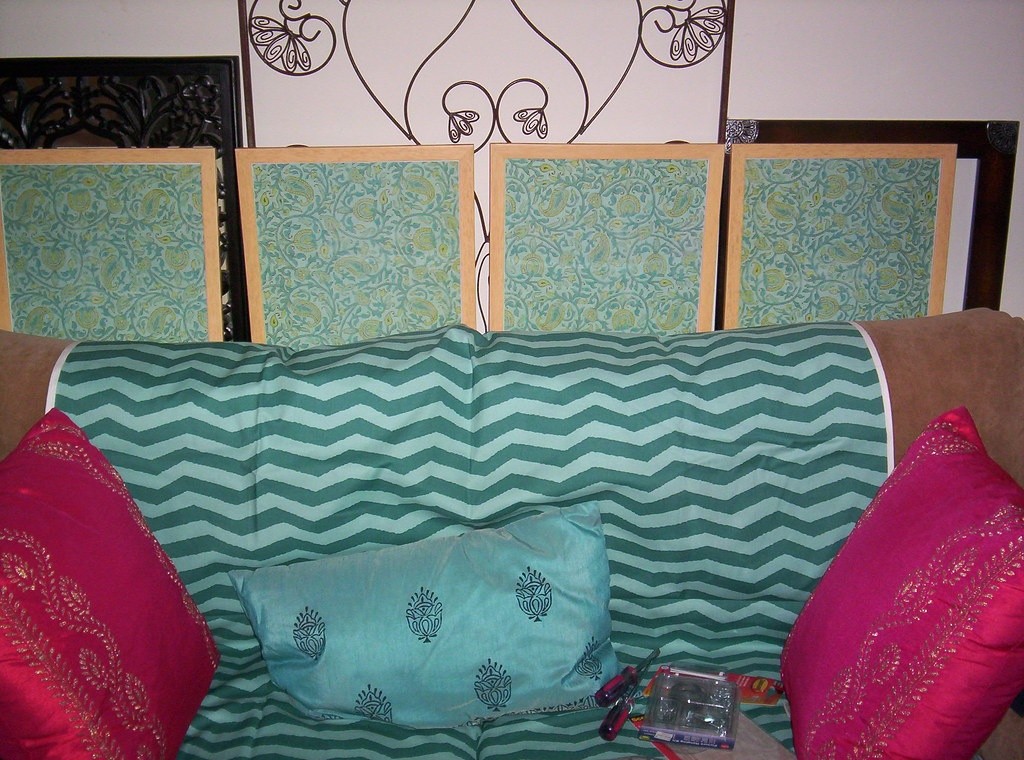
[597,659,655,742]
[595,647,662,707]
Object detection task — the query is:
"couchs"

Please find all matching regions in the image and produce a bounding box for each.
[0,307,1023,760]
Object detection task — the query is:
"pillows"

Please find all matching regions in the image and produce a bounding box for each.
[0,409,220,760]
[779,404,1024,760]
[226,501,618,725]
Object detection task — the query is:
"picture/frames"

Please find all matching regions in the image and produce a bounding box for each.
[724,143,958,330]
[1,148,223,342]
[236,144,476,352]
[490,143,725,337]
